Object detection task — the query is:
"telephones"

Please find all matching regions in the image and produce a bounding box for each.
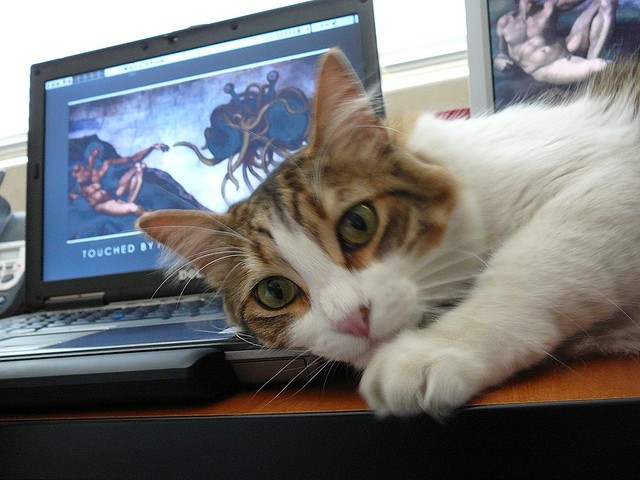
[0,197,28,318]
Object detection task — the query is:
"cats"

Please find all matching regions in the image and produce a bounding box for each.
[134,45,640,425]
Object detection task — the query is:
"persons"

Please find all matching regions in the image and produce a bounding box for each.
[65,142,172,223]
[492,0,616,87]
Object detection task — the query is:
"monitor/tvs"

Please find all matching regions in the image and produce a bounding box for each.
[466,2,639,123]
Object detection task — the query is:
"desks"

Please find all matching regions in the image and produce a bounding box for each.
[0,355,639,480]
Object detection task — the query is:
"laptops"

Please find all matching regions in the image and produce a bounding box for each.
[1,1,387,419]
